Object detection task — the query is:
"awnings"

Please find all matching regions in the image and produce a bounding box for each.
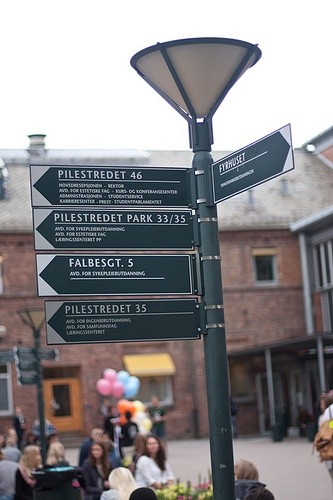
[123,352,177,378]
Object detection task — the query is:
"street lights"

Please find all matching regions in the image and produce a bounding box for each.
[128,37,262,499]
[16,307,49,472]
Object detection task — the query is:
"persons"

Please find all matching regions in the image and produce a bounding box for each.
[316,390,333,481]
[0,394,168,500]
[234,460,276,500]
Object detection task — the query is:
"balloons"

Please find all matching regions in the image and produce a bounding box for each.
[97,369,152,435]
